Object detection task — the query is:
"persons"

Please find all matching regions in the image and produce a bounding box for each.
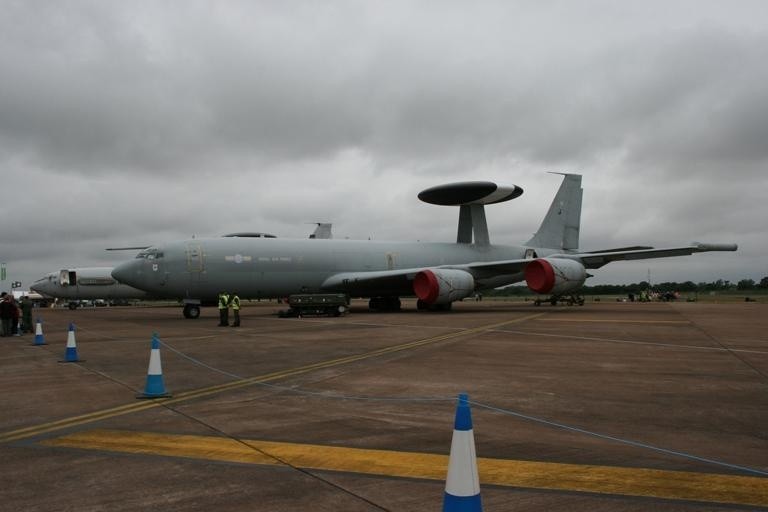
[474,292,482,301]
[640,289,681,302]
[0,292,34,336]
[217,289,240,327]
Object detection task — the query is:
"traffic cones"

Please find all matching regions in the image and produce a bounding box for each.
[30,323,44,345]
[65,323,79,362]
[442,393,483,511]
[143,334,167,395]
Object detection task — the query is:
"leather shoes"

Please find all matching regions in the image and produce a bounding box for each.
[218,324,240,327]
